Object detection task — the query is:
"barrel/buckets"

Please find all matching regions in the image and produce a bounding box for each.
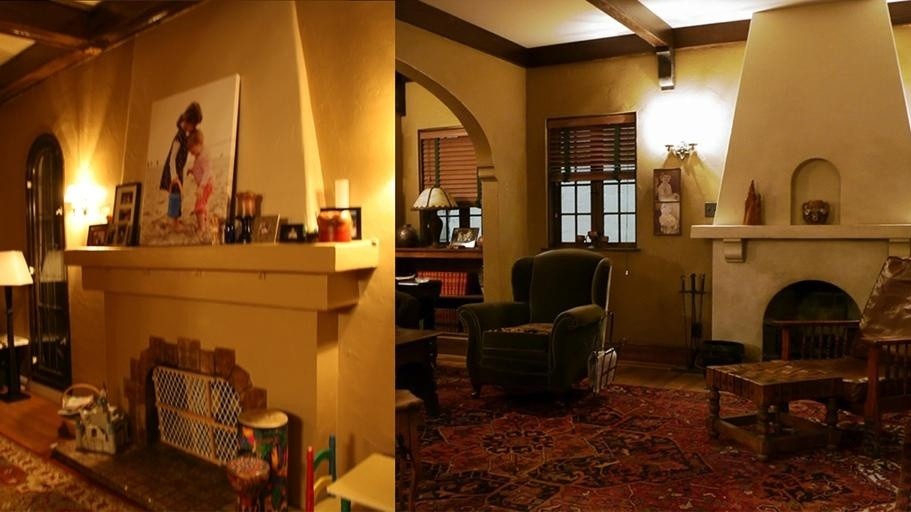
[700,340,744,378]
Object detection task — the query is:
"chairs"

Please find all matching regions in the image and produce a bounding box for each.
[769,256,910,447]
[304,434,367,511]
[396,291,420,330]
[456,249,616,409]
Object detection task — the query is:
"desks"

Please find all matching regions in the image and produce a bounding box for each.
[1,333,29,352]
[326,450,395,512]
[395,328,445,417]
[397,278,442,368]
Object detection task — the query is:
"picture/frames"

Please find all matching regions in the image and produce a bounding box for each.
[85,181,141,247]
[650,165,682,236]
[250,207,362,246]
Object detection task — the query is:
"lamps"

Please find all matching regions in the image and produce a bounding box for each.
[0,250,35,404]
[410,187,460,247]
[40,249,64,285]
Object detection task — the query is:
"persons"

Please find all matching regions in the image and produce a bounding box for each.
[657,171,680,201]
[186,128,215,235]
[159,100,203,227]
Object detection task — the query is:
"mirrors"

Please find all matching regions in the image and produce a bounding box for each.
[24,131,74,395]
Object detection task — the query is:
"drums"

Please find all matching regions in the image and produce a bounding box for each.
[238,408,290,512]
[228,455,270,512]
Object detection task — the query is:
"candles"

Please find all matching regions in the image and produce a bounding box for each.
[334,178,350,210]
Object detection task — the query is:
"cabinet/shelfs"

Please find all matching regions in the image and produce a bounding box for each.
[393,246,488,357]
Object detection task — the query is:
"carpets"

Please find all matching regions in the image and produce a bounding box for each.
[0,425,163,512]
[395,382,910,511]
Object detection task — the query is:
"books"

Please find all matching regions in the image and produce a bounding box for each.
[417,271,468,333]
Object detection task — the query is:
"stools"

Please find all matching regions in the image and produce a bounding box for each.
[395,387,426,472]
[704,361,844,464]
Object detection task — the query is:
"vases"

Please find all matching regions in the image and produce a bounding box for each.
[396,224,416,247]
